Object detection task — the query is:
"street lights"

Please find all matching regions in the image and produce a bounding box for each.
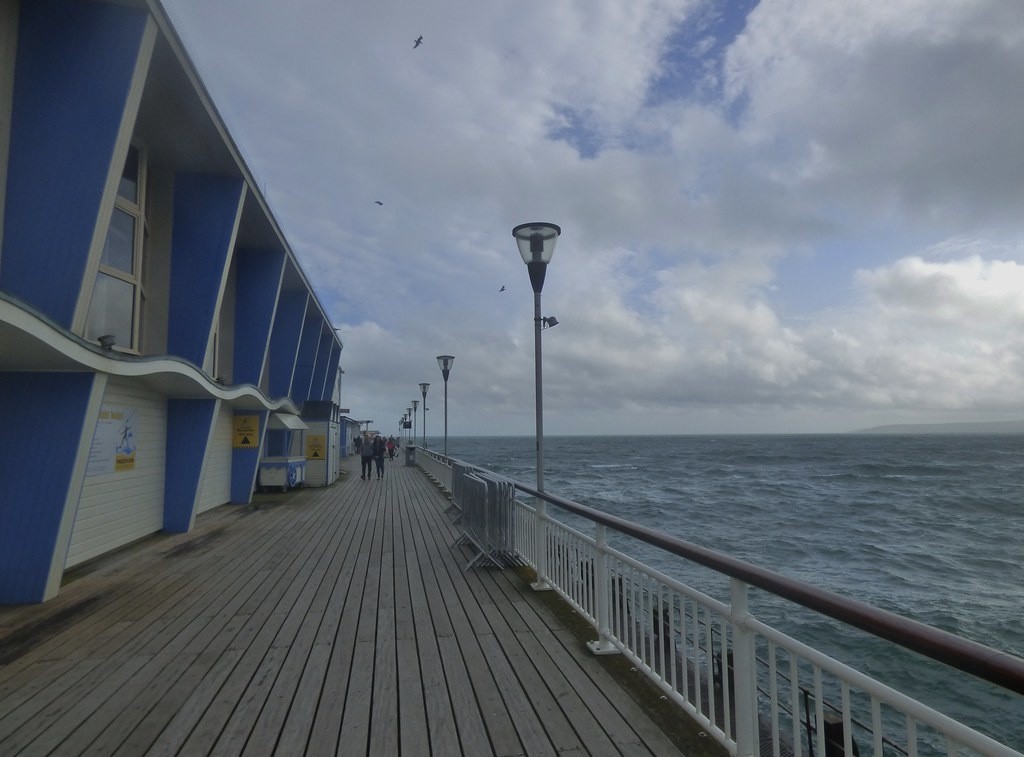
[418,383,430,449]
[436,355,455,457]
[398,413,408,443]
[411,400,419,446]
[512,221,562,494]
[407,407,413,441]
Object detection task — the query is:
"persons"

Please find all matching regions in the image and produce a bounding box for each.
[360,435,374,481]
[371,436,386,480]
[355,435,396,461]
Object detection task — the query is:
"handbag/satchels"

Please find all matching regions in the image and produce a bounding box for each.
[393,448,400,457]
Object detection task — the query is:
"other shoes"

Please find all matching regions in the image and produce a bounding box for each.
[367,475,371,480]
[360,475,366,480]
